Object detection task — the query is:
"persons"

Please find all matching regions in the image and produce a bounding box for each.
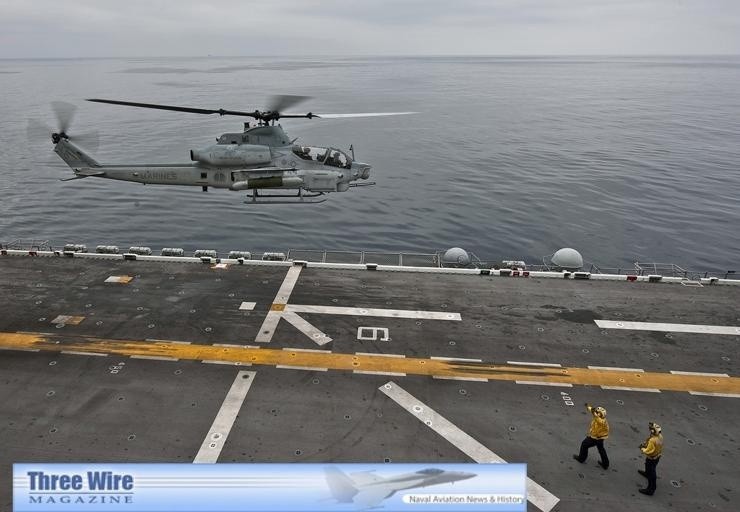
[573,402,610,469]
[638,422,663,495]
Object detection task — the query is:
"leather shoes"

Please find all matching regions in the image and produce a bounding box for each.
[572,454,584,463]
[638,489,654,496]
[598,460,608,470]
[637,469,646,478]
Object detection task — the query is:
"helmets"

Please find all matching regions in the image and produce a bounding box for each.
[595,407,607,418]
[649,422,662,436]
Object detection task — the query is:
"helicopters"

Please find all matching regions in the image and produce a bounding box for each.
[28,97,418,207]
